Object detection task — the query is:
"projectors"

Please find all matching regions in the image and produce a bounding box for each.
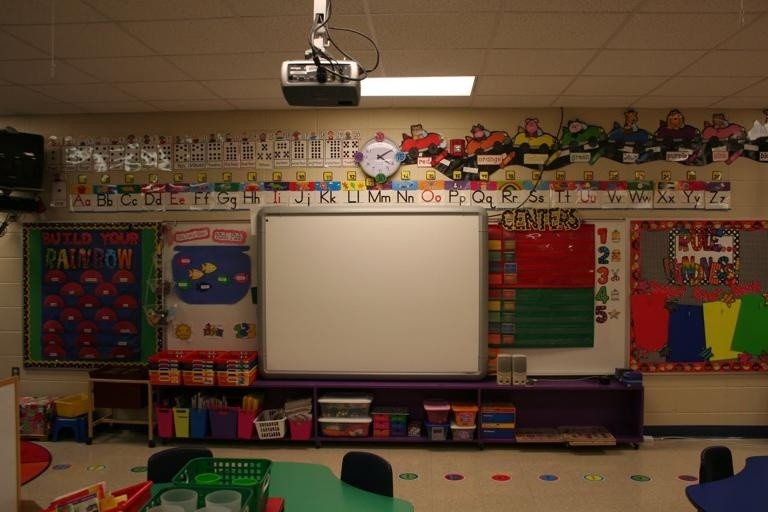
[280,60,360,106]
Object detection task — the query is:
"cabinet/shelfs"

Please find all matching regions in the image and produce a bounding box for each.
[152,373,644,450]
[86,378,157,447]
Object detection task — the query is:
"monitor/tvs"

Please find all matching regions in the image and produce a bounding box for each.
[0,129,44,191]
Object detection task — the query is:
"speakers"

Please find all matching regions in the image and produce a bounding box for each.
[512,354,526,386]
[497,354,512,386]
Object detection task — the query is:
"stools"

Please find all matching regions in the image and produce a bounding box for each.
[47,418,85,443]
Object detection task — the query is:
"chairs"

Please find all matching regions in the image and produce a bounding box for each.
[341,451,393,497]
[147,447,213,484]
[699,446,734,484]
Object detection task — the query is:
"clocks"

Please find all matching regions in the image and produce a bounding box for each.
[359,137,401,177]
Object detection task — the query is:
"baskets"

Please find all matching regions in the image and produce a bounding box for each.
[137,457,273,512]
[253,409,287,440]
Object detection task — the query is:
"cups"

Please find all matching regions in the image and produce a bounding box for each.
[191,471,260,485]
[146,488,242,511]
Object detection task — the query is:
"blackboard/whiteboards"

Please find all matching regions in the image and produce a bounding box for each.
[256,205,489,380]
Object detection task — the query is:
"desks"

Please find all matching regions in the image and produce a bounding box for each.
[685,456,768,512]
[150,459,414,512]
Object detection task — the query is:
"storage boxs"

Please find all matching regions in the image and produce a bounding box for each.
[425,421,449,441]
[317,397,373,417]
[423,401,450,423]
[208,408,237,440]
[452,403,479,426]
[450,421,477,441]
[318,419,373,438]
[289,419,312,440]
